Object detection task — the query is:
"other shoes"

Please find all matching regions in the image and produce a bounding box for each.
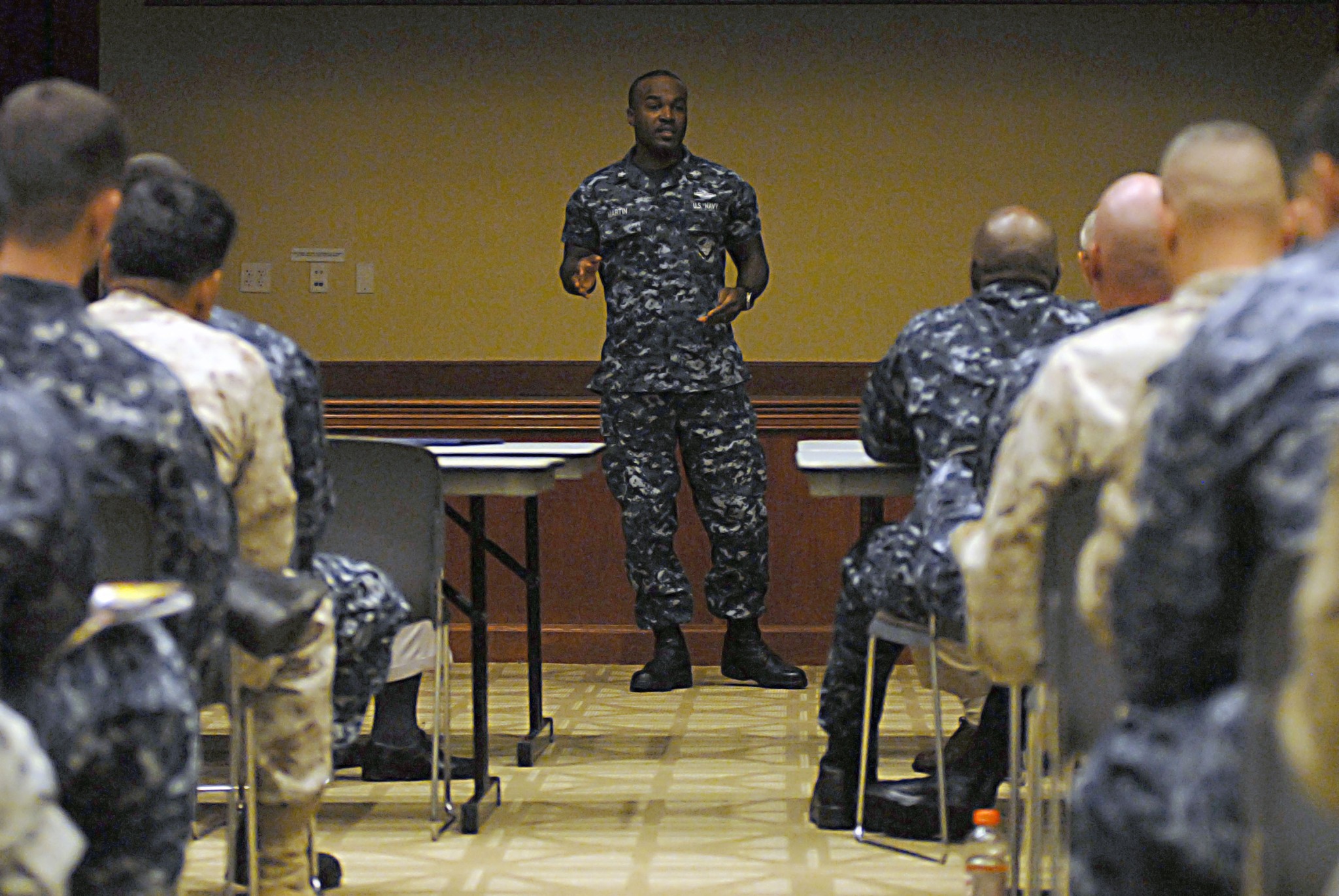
[363,727,474,783]
[330,733,372,768]
[911,716,980,773]
[225,814,341,891]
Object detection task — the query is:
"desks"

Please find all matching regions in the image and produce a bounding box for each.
[427,443,614,767]
[430,455,568,835]
[795,440,922,530]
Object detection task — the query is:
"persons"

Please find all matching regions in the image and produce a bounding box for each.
[559,73,807,688]
[0,79,476,895]
[810,72,1339,896]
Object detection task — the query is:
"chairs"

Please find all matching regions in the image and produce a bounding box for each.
[193,432,1339,896]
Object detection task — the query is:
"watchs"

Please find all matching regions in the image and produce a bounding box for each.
[736,283,755,311]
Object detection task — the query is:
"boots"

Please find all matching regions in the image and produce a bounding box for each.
[630,624,692,693]
[808,736,878,830]
[721,617,808,690]
[863,720,1006,843]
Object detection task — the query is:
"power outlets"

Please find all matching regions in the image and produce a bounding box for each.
[240,262,274,294]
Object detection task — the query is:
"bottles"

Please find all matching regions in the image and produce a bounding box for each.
[964,807,1013,896]
